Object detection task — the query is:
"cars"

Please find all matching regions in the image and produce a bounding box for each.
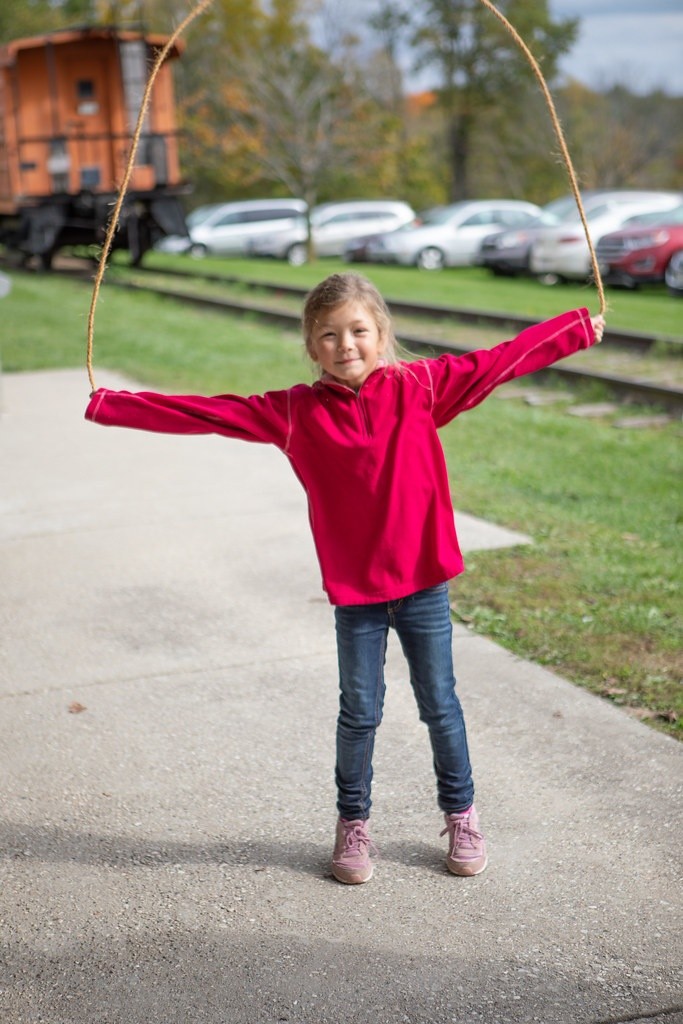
[526,191,683,281]
[466,189,618,271]
[343,205,445,264]
[366,200,564,271]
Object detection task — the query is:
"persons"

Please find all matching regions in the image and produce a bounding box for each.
[84,273,609,886]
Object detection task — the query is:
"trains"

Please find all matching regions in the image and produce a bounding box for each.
[0,19,196,276]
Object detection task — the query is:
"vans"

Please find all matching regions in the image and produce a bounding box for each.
[151,192,311,269]
[247,199,416,266]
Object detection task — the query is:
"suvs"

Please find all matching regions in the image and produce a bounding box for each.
[594,202,682,296]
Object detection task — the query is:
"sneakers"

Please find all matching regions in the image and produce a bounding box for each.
[331,816,378,884]
[440,804,488,875]
